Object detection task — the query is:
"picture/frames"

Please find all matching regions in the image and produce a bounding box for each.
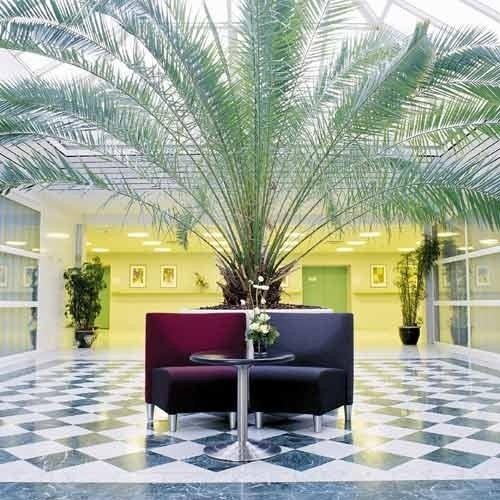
[159,264,177,288]
[475,264,489,287]
[370,263,387,287]
[129,264,147,288]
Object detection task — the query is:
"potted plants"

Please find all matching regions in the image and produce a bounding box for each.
[441,239,473,346]
[29,265,39,349]
[63,255,107,347]
[394,233,440,345]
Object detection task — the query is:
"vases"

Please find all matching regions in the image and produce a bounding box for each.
[251,337,267,357]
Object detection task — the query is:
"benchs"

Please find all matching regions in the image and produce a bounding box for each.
[145,309,355,431]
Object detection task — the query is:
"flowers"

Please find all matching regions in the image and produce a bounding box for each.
[245,313,281,345]
